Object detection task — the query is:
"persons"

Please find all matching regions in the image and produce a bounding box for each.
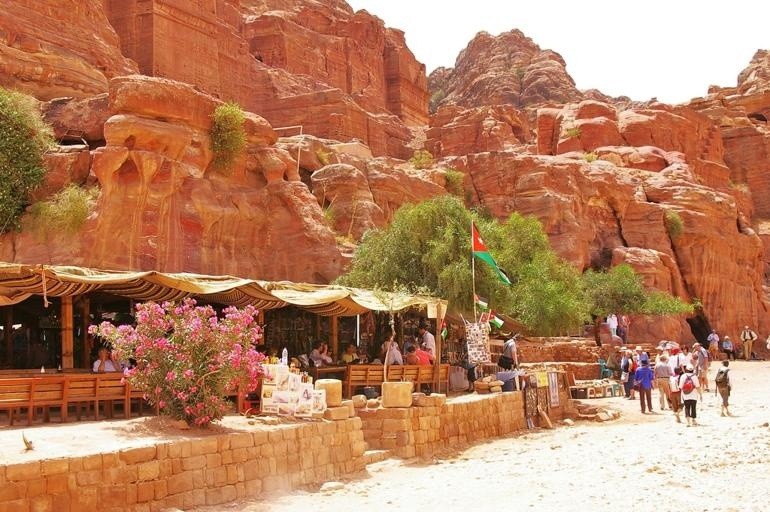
[496,332,522,370]
[765,334,770,351]
[340,341,359,362]
[722,335,737,360]
[715,359,734,418]
[268,345,279,356]
[739,325,758,361]
[378,324,436,365]
[467,363,484,394]
[92,347,132,374]
[255,344,267,356]
[707,329,720,362]
[606,311,713,427]
[307,339,334,368]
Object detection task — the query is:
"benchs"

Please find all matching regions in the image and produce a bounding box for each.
[223,363,450,418]
[0,368,160,425]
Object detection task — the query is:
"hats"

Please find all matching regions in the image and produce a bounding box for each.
[655,343,680,355]
[619,346,642,352]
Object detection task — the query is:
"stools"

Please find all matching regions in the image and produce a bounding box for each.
[582,384,624,399]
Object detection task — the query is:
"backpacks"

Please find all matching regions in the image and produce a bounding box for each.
[682,373,696,394]
[714,368,730,388]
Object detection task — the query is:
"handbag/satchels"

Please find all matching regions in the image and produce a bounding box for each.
[499,353,513,370]
[633,380,643,391]
[621,371,629,382]
[700,348,713,362]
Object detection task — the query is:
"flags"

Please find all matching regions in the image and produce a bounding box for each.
[473,221,512,287]
[439,293,506,342]
